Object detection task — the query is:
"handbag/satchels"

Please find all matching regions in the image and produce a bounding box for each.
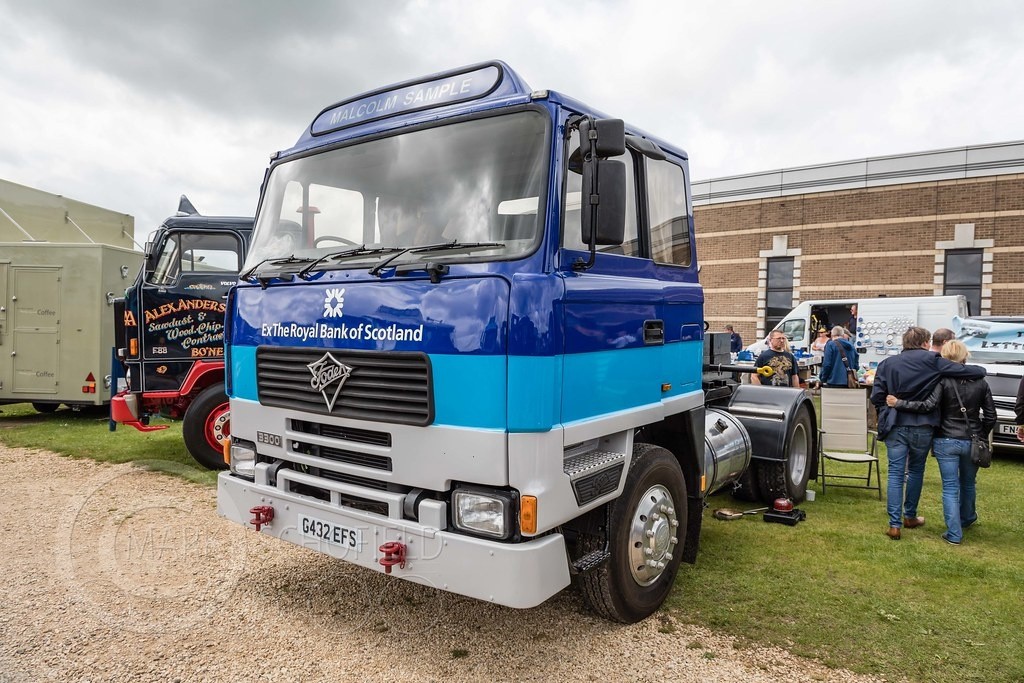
[971,436,991,468]
[847,369,860,389]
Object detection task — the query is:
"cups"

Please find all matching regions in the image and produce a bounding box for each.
[806,489,816,502]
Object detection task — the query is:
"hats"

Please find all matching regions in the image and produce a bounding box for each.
[818,327,827,333]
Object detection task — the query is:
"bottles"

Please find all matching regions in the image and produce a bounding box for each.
[142,413,150,425]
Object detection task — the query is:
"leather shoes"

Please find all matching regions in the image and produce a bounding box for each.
[903,516,925,528]
[886,527,901,540]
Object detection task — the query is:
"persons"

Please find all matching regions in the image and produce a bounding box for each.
[813,326,860,388]
[751,331,800,388]
[724,324,743,353]
[1014,373,1024,442]
[872,327,997,545]
[847,304,857,336]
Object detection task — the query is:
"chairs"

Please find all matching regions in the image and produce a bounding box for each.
[817,387,882,501]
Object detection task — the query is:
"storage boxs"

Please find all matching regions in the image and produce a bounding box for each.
[799,369,812,379]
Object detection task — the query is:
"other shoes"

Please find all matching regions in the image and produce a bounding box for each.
[962,513,977,528]
[942,532,960,546]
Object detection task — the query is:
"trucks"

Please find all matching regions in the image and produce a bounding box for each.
[0,178,236,420]
[214,57,825,627]
[742,294,969,390]
[944,314,1024,456]
[104,193,364,473]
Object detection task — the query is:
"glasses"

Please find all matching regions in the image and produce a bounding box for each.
[772,336,785,339]
